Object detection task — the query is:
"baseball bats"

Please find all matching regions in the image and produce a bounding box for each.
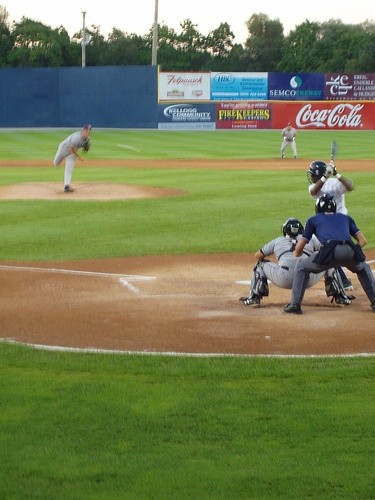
[328,139,336,176]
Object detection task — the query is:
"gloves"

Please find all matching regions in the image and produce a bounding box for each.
[320,160,341,183]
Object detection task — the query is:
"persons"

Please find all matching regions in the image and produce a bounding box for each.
[243,218,351,307]
[282,193,375,314]
[281,122,297,160]
[53,123,92,192]
[306,161,355,291]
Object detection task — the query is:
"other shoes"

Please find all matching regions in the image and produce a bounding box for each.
[284,304,303,314]
[63,188,74,192]
[342,281,354,291]
[335,298,352,305]
[243,293,261,308]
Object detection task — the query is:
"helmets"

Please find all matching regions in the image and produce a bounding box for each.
[315,193,337,213]
[305,159,328,179]
[283,217,305,237]
